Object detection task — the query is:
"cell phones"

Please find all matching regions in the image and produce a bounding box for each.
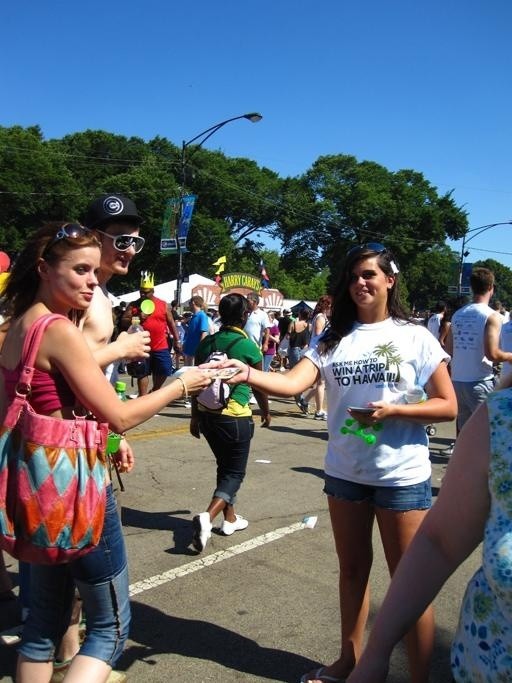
[349,404,379,417]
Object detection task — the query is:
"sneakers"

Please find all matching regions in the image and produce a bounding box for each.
[298,399,311,415]
[220,514,250,537]
[191,511,214,556]
[314,411,329,420]
[126,392,140,400]
[280,367,286,374]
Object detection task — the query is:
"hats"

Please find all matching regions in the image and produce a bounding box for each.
[85,195,145,229]
[283,308,293,316]
[140,270,155,290]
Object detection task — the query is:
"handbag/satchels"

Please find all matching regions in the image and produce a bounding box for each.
[1,312,111,568]
[277,329,291,360]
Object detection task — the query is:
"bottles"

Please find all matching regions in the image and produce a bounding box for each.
[405,382,430,426]
[106,381,128,453]
[128,316,145,362]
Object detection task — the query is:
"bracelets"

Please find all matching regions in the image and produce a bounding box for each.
[176,377,189,399]
[243,364,250,384]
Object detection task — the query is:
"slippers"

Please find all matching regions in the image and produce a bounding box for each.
[299,667,340,683]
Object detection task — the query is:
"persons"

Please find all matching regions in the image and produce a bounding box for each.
[170,313,192,373]
[278,308,293,369]
[297,294,334,421]
[439,296,469,377]
[285,307,313,401]
[444,267,512,439]
[120,285,182,398]
[242,292,270,404]
[496,310,512,390]
[198,243,459,682]
[169,300,183,370]
[427,300,450,348]
[191,293,271,554]
[57,194,152,683]
[263,312,280,372]
[499,306,510,320]
[492,299,509,324]
[1,221,213,683]
[178,296,209,366]
[344,388,512,683]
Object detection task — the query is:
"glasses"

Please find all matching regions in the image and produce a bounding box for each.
[98,228,147,254]
[50,221,92,247]
[345,243,388,255]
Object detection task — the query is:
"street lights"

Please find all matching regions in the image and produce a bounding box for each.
[173,112,263,309]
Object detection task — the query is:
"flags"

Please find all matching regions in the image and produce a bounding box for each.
[258,258,271,288]
[211,256,226,284]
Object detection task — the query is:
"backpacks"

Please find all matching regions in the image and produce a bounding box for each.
[195,333,246,414]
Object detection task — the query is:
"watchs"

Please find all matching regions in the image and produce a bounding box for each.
[174,340,181,343]
